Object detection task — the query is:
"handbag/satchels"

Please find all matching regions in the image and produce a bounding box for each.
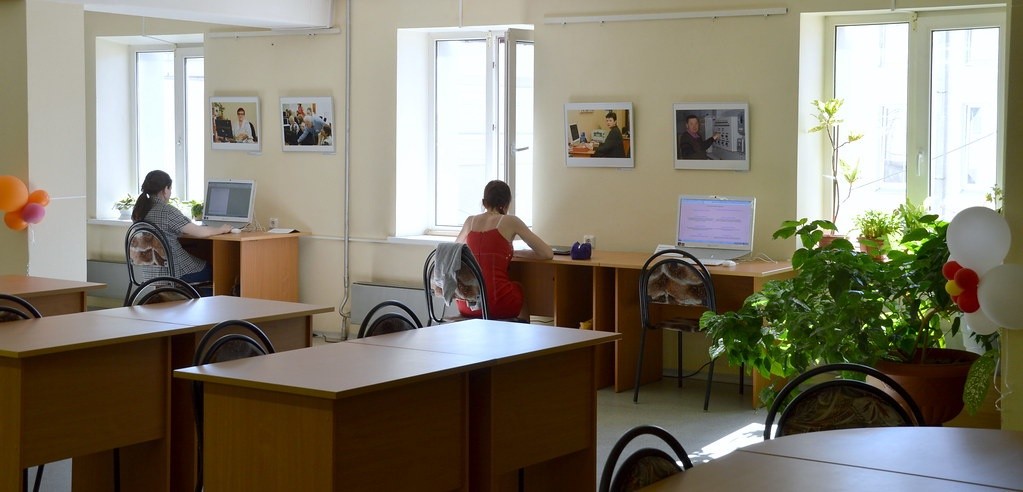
[570,241,592,261]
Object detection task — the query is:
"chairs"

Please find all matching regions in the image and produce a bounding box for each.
[0,294,44,492]
[423,243,527,326]
[633,250,744,412]
[763,363,926,440]
[123,221,213,307]
[357,300,423,338]
[191,318,277,492]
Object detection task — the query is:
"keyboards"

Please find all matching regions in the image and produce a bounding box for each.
[676,257,725,266]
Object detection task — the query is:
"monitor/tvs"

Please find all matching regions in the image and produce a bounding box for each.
[676,195,756,261]
[202,178,257,230]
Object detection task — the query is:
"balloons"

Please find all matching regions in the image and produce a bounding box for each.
[0,174,50,231]
[942,206,1023,335]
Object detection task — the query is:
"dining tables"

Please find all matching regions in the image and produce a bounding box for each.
[598,425,694,492]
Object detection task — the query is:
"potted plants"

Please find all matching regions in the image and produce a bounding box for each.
[182,200,204,221]
[112,193,136,220]
[807,97,865,248]
[698,181,1003,423]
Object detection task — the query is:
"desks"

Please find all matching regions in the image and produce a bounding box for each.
[156,232,313,303]
[0,275,625,492]
[511,249,800,410]
[633,427,1023,492]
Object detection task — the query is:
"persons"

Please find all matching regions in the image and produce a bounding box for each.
[680,115,721,160]
[454,179,554,325]
[285,103,332,145]
[232,108,253,142]
[592,113,626,158]
[131,170,234,298]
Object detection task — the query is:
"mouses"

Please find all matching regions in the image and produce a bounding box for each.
[724,260,736,267]
[231,228,241,233]
